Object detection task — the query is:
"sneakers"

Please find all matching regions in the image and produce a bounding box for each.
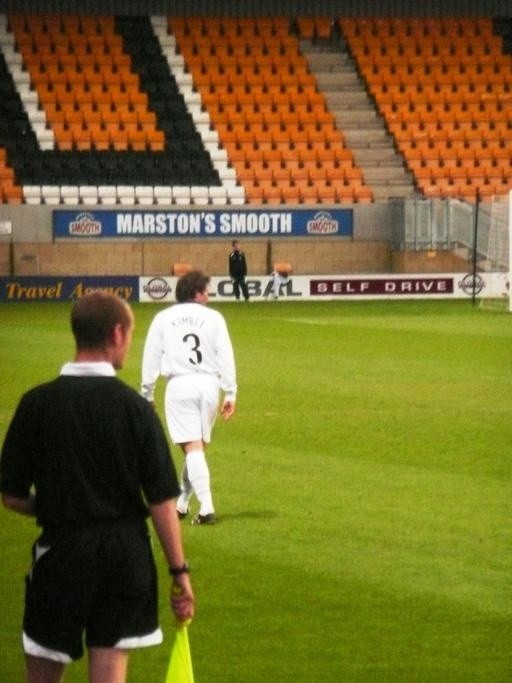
[176,509,215,525]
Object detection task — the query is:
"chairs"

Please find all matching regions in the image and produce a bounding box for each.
[340,17,512,198]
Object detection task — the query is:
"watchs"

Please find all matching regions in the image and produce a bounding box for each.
[167,561,189,576]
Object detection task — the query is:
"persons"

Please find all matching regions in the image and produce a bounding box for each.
[0,291,195,683]
[229,239,249,302]
[141,270,237,526]
[271,267,289,299]
